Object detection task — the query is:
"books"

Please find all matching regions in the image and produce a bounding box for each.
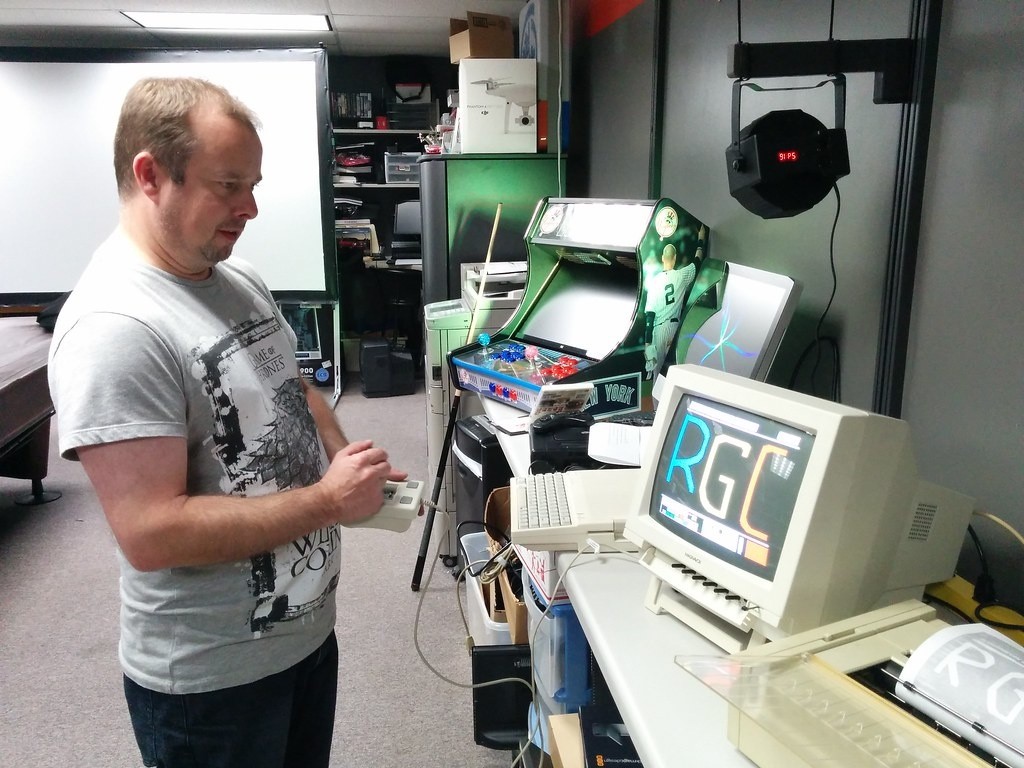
[333,165,372,183]
[335,218,371,224]
[330,90,372,118]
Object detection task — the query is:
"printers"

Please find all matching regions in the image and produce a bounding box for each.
[669,596,1024,768]
[424,261,529,556]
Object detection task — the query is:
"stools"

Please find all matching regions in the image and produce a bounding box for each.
[378,269,422,347]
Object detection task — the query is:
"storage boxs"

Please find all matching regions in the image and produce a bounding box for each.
[484,485,529,646]
[375,116,387,129]
[520,568,565,696]
[458,59,538,153]
[460,532,513,646]
[386,142,398,153]
[384,151,422,185]
[509,542,571,606]
[580,703,644,768]
[548,712,585,768]
[387,101,436,131]
[446,89,460,106]
[448,11,515,64]
[342,331,409,372]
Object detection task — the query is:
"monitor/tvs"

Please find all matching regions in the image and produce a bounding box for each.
[394,199,421,236]
[621,363,976,658]
[516,260,638,360]
[359,337,416,398]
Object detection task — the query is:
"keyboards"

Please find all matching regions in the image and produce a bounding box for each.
[517,472,573,530]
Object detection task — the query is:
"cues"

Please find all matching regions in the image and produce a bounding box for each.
[410,201,504,594]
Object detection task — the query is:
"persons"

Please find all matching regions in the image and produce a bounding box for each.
[645,223,706,410]
[48,77,408,768]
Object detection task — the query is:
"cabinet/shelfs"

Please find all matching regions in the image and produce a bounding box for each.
[527,573,592,714]
[332,128,435,188]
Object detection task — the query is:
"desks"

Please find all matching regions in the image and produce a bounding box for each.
[0,364,62,505]
[572,551,761,768]
